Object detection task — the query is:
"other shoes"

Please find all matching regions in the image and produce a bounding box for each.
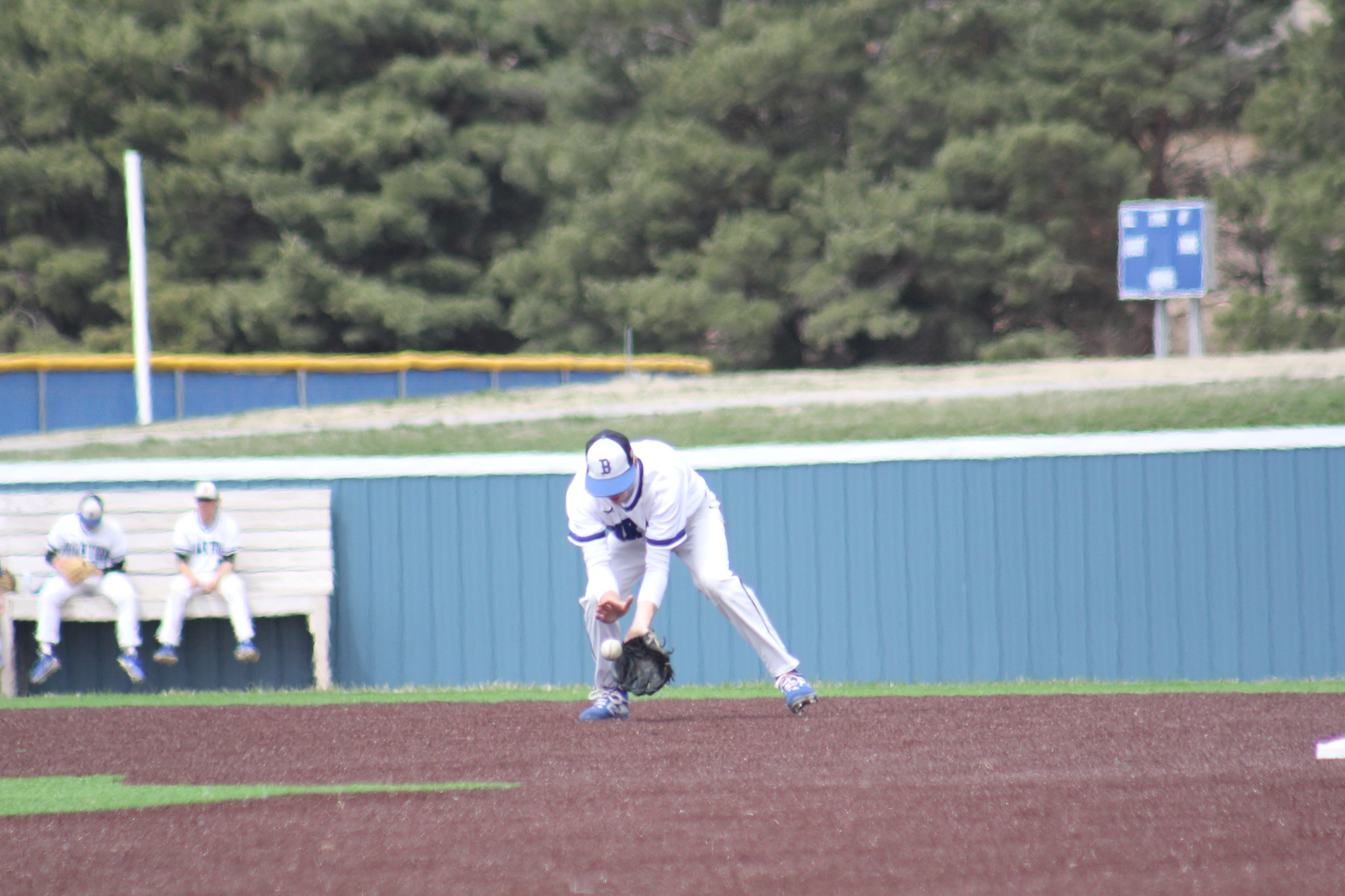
[29,648,60,684]
[154,646,179,663]
[116,653,145,681]
[235,642,261,662]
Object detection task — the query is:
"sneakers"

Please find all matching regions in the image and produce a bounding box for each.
[578,689,630,721]
[775,673,819,717]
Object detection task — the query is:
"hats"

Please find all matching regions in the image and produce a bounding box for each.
[583,429,635,498]
[77,494,103,527]
[194,480,218,500]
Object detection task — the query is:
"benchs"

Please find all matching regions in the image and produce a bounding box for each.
[0,492,335,687]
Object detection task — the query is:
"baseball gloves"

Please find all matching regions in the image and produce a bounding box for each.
[610,630,673,697]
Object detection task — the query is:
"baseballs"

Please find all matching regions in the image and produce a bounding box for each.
[600,638,622,661]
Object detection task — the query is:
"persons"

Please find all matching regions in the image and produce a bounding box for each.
[27,494,143,684]
[563,428,818,722]
[154,480,260,665]
